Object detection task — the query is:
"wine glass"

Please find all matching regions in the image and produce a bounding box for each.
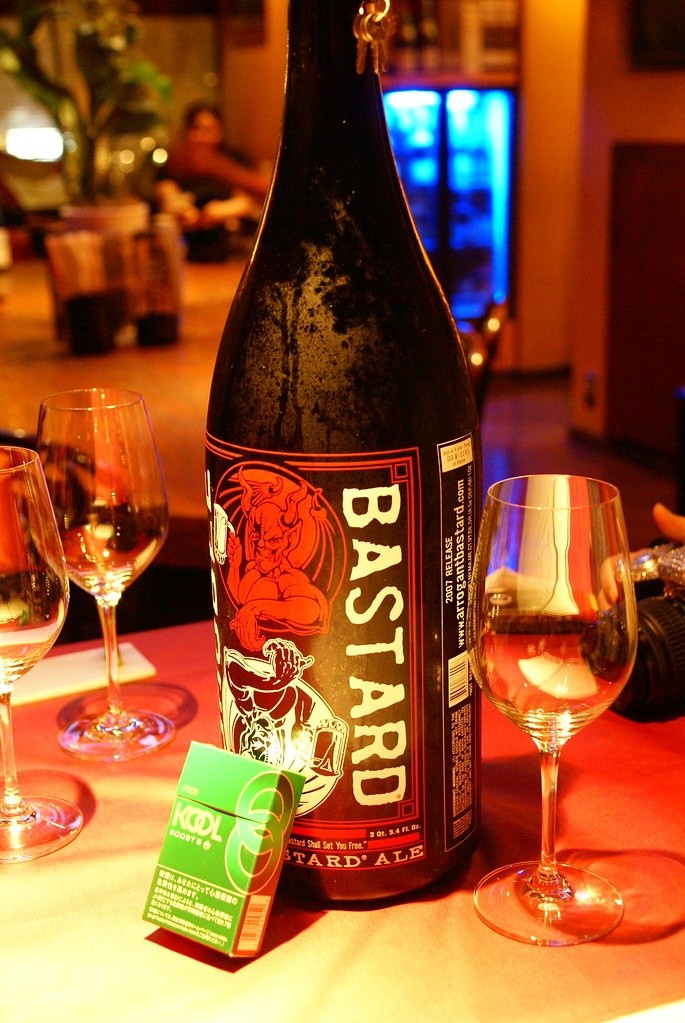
[29,389,175,761]
[0,446,84,864]
[466,473,639,948]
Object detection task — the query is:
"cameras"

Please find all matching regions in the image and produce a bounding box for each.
[580,590,685,725]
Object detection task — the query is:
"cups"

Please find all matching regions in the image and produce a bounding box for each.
[47,233,130,355]
[123,227,184,348]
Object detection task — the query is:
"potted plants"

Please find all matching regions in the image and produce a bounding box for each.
[0,0,175,230]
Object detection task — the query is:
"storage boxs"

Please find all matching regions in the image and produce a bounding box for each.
[141,743,306,960]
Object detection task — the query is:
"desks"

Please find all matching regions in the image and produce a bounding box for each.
[0,589,685,1022]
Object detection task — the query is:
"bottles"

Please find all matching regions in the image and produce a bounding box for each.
[203,0,484,906]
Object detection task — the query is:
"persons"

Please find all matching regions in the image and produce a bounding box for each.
[149,104,254,263]
